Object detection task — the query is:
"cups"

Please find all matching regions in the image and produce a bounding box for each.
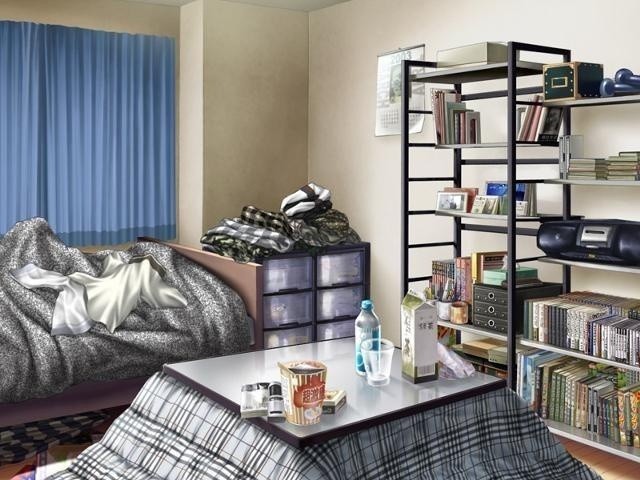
[279,360,327,425]
[451,302,469,325]
[359,338,394,387]
[439,300,454,321]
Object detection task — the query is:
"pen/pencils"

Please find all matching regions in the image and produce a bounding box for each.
[443,280,459,302]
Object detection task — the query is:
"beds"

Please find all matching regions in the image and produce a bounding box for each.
[0,236,264,429]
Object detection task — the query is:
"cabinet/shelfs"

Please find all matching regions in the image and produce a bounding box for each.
[201,239,372,349]
[507,41,640,462]
[400,41,573,437]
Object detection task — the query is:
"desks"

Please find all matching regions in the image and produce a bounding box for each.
[162,338,508,450]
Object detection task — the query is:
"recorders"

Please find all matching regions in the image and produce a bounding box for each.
[537,220,640,266]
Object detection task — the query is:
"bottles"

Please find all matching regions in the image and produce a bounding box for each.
[354,300,382,376]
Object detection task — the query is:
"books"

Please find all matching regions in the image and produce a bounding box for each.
[516,348,639,449]
[523,289,639,368]
[516,95,564,147]
[429,88,481,144]
[430,250,508,325]
[558,135,640,182]
